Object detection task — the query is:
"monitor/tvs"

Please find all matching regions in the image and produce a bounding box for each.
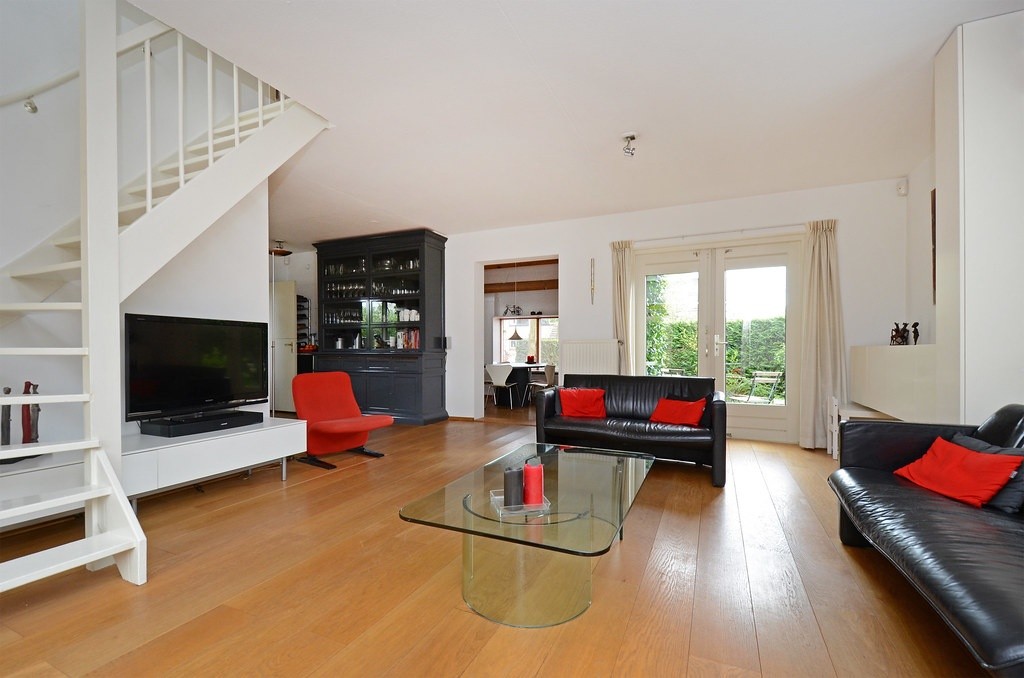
[124,313,268,422]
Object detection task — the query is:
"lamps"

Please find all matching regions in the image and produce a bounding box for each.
[621,131,638,158]
[270,239,292,258]
[507,263,523,341]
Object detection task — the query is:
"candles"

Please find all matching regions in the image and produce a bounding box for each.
[502,466,524,512]
[524,464,544,505]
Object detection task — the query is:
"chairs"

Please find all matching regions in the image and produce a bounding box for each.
[731,371,784,404]
[521,364,556,407]
[484,363,520,411]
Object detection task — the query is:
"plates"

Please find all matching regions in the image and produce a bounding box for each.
[525,362,536,364]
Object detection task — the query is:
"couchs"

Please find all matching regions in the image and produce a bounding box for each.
[827,405,1024,677]
[534,373,727,487]
[292,372,395,469]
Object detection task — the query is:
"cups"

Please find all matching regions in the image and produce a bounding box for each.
[324,312,339,324]
[389,288,420,294]
[398,308,420,321]
[524,464,543,508]
[503,466,523,512]
[527,355,534,362]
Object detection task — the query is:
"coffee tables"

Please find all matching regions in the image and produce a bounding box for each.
[398,443,656,628]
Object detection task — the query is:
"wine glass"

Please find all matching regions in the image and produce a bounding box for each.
[337,307,361,324]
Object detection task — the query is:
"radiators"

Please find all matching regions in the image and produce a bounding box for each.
[559,337,622,385]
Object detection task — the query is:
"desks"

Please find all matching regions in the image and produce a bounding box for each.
[118,416,307,518]
[500,363,546,409]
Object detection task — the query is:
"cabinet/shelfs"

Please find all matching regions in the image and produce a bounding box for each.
[314,226,448,426]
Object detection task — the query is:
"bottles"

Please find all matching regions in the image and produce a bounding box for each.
[297,294,307,347]
[372,281,385,295]
[325,282,366,298]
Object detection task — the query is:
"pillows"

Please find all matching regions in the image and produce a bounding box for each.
[953,434,1023,515]
[889,436,1024,508]
[648,397,706,427]
[555,385,590,415]
[667,390,713,428]
[559,387,607,418]
